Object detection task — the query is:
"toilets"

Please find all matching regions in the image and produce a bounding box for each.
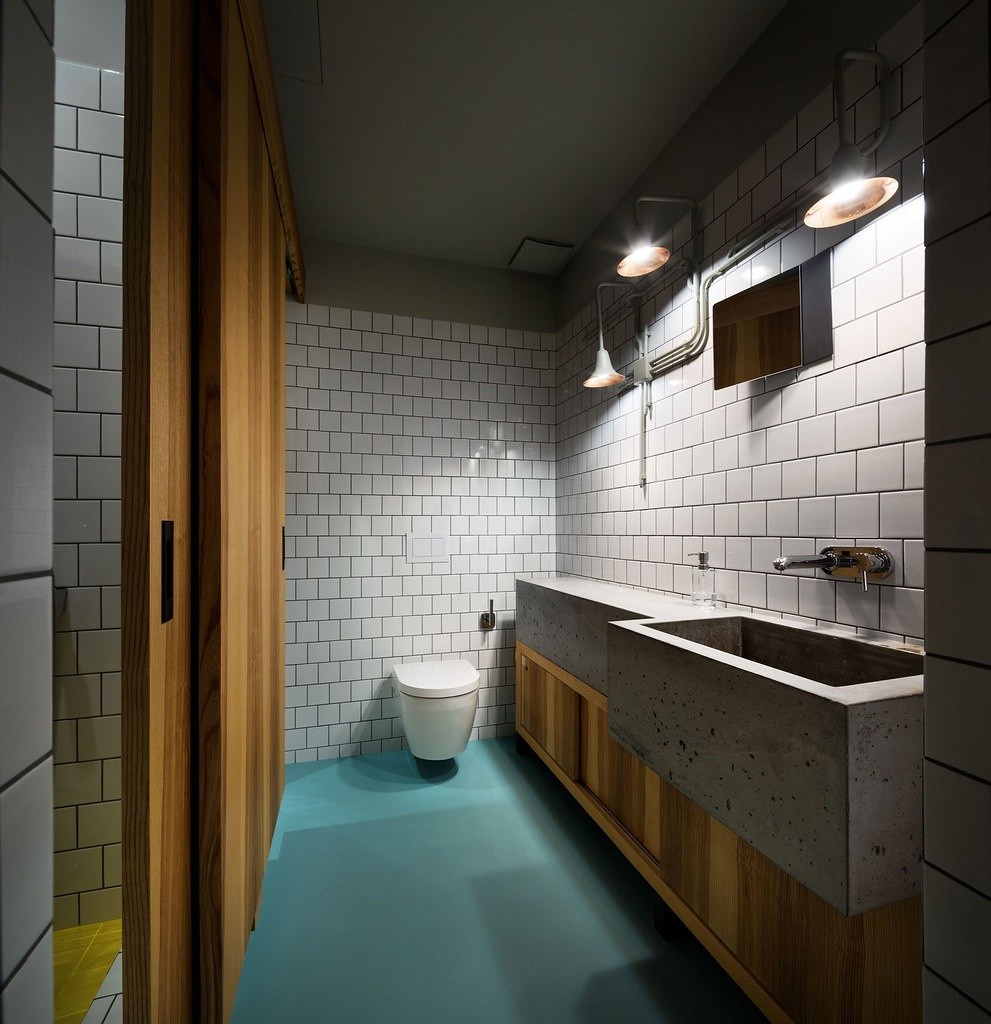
[392,659,481,763]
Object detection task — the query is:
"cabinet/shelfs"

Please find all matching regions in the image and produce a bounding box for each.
[515,638,924,1024]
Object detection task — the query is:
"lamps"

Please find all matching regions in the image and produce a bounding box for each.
[802,143,899,229]
[616,224,670,278]
[583,350,626,388]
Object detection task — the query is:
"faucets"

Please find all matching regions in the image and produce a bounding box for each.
[772,550,841,572]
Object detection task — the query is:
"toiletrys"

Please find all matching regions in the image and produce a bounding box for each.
[687,551,715,608]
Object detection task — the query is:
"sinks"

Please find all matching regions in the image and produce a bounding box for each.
[605,606,925,919]
